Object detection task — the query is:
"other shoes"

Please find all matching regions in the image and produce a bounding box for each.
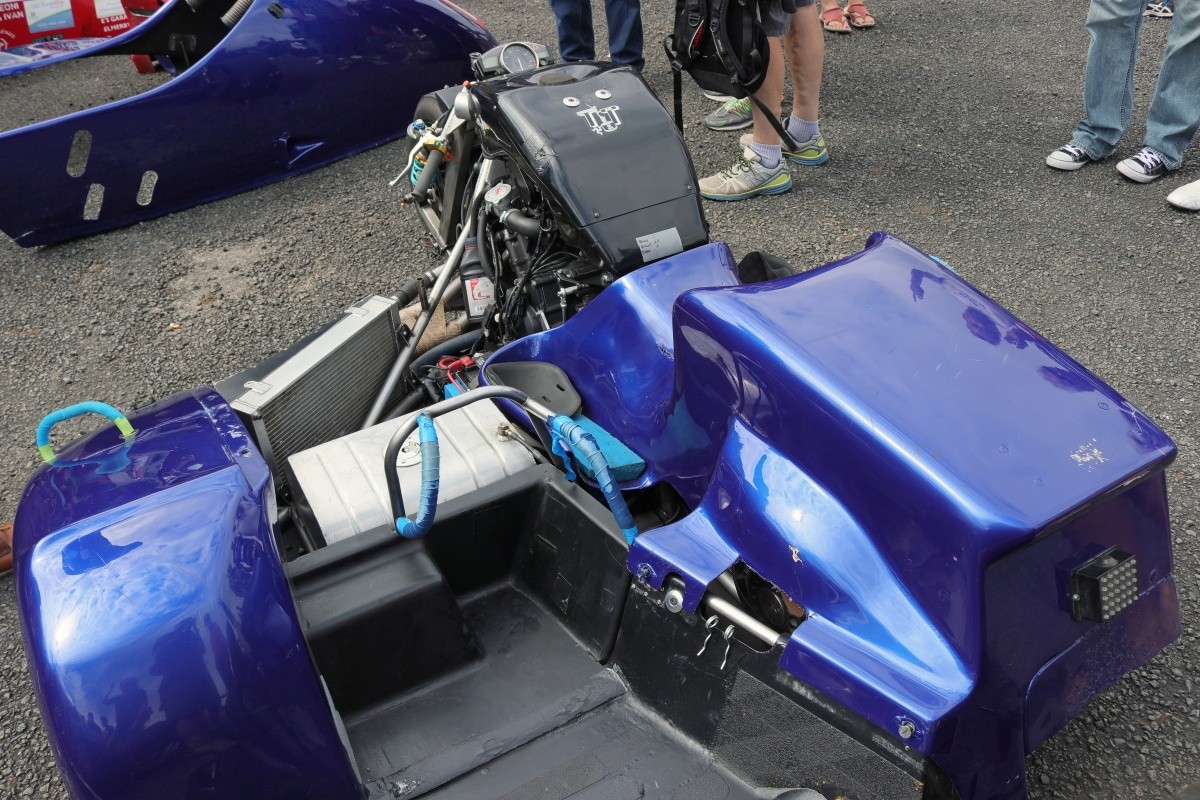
[1166,179,1200,210]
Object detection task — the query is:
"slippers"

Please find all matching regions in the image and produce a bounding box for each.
[844,4,875,27]
[819,8,851,32]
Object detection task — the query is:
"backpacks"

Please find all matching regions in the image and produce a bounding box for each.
[662,0,798,153]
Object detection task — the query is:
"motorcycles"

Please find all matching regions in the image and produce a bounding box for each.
[12,1,1181,798]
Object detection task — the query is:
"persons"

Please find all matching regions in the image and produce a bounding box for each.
[1045,0,1200,183]
[549,0,645,75]
[1142,0,1174,18]
[697,0,877,203]
[1165,179,1200,211]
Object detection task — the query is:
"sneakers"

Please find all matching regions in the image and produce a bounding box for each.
[1141,0,1174,17]
[1046,143,1093,170]
[704,96,754,130]
[698,145,793,200]
[1116,146,1167,183]
[739,117,829,165]
